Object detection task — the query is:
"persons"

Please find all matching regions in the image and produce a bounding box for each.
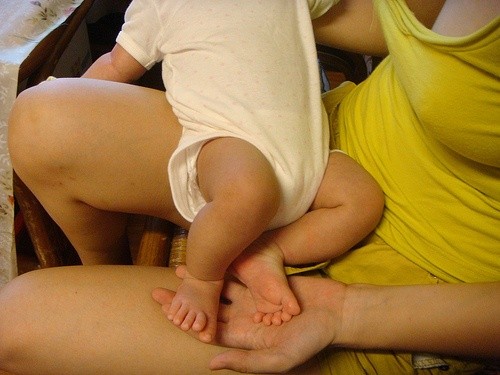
[38,0,384,342]
[0,0,500,375]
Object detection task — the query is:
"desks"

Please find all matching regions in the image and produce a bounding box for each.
[0,0,132,287]
